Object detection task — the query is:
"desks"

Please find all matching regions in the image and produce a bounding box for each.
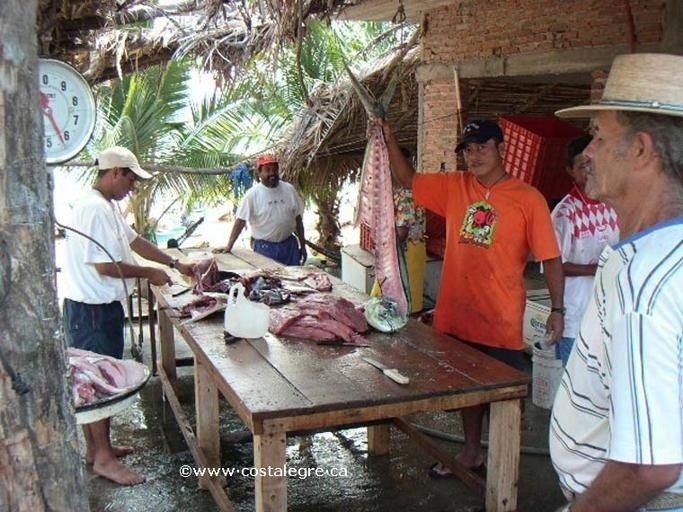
[126,250,533,510]
[339,246,442,311]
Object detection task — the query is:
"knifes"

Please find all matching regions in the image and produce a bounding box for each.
[168,280,203,294]
[360,354,408,385]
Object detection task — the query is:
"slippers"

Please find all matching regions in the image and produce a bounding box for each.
[428,460,485,479]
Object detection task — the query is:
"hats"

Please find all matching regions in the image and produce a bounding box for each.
[96,146,154,180]
[454,121,503,153]
[554,52,683,123]
[257,156,279,169]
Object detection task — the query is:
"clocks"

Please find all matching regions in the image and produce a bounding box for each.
[34,55,98,168]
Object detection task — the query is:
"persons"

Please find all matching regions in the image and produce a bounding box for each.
[364,115,565,479]
[549,53,683,512]
[212,154,307,266]
[541,135,619,367]
[60,146,196,486]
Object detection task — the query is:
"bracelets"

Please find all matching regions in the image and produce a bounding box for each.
[551,307,566,315]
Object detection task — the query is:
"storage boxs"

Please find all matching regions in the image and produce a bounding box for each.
[359,210,446,254]
[521,286,565,352]
[495,114,584,199]
[424,231,445,259]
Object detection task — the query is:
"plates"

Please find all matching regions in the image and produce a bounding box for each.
[187,250,215,261]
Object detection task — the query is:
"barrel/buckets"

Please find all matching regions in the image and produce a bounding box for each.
[532,339,563,409]
[223,283,271,340]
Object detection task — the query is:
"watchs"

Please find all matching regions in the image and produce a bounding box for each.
[169,257,179,268]
